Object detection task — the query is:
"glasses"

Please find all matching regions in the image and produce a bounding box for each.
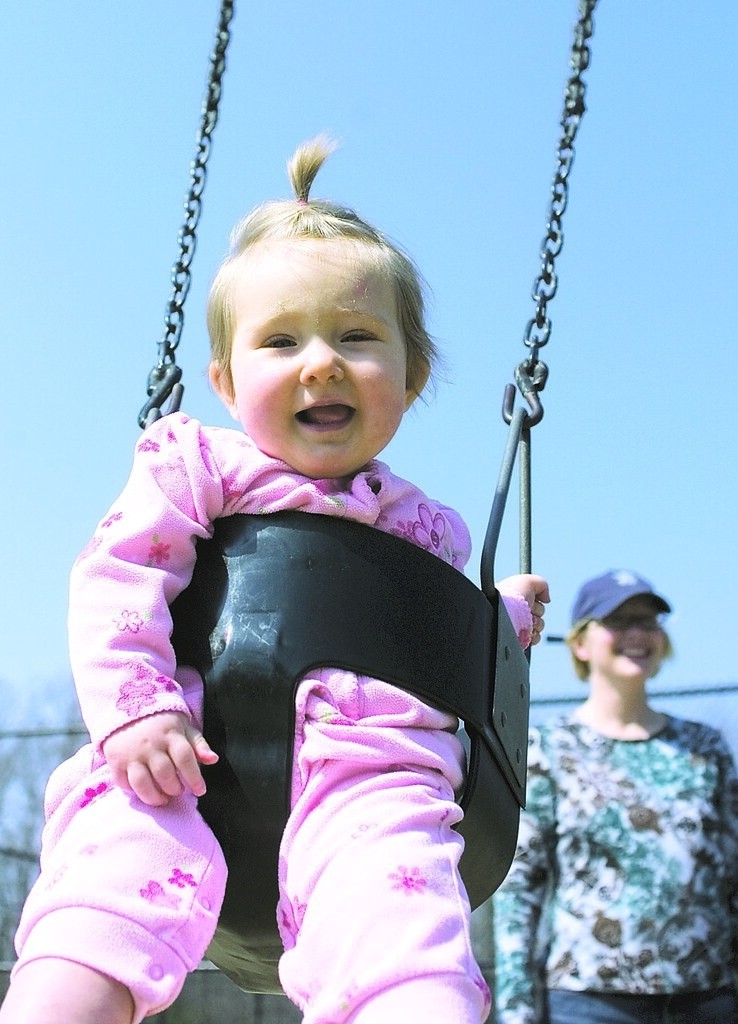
[592,613,662,631]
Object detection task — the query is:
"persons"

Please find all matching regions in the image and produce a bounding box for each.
[492,571,738,1024]
[1,135,550,1024]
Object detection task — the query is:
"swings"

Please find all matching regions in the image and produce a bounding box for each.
[138,0,600,995]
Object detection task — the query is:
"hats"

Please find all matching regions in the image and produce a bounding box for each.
[571,570,671,626]
